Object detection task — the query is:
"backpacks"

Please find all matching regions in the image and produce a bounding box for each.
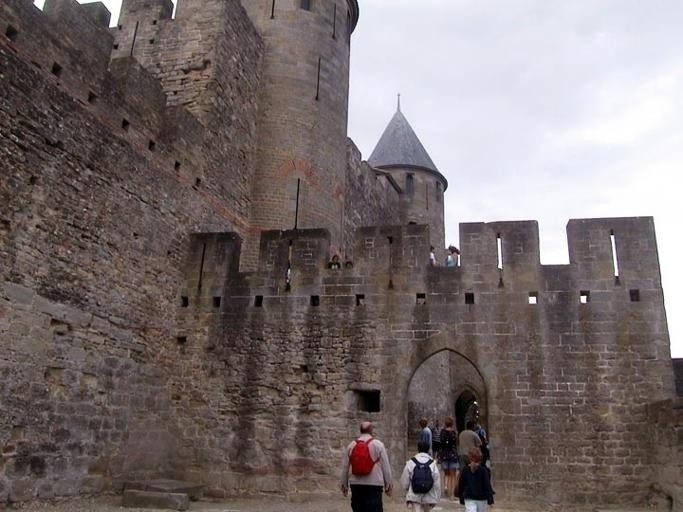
[440,430,455,451]
[411,456,434,493]
[351,438,379,475]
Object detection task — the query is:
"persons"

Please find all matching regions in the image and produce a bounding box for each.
[398,441,443,512]
[337,421,395,512]
[416,415,489,502]
[457,447,495,512]
